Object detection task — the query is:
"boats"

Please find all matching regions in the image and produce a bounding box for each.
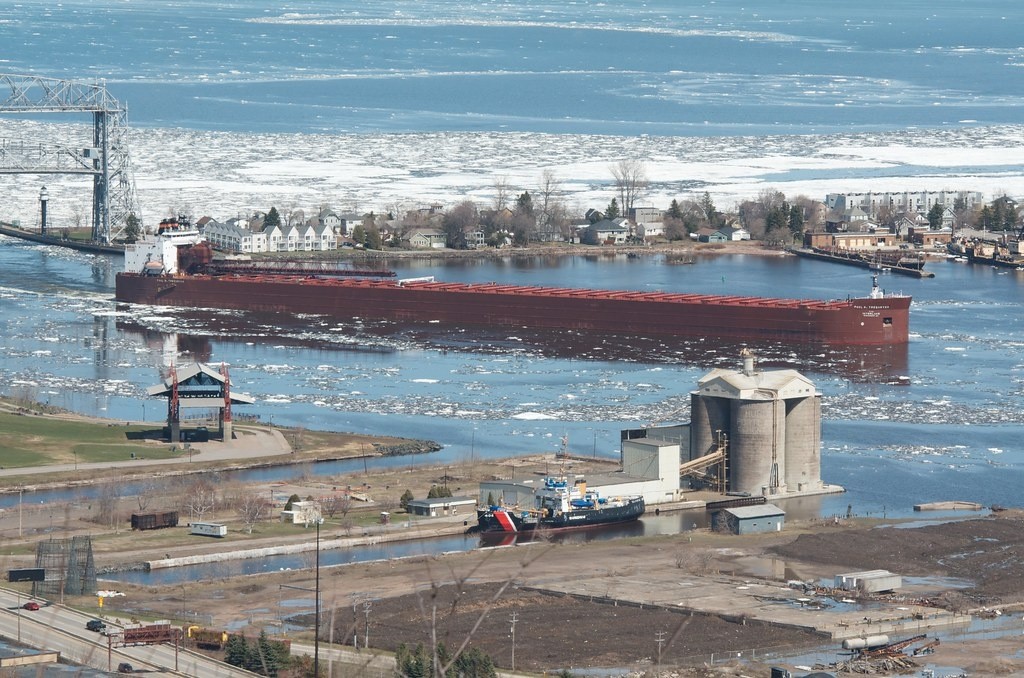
[474,459,648,535]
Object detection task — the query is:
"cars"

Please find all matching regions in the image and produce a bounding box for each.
[87,620,107,633]
[23,603,40,611]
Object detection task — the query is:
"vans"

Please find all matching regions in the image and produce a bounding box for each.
[119,663,133,672]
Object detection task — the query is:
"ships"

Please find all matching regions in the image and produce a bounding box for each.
[113,204,916,351]
